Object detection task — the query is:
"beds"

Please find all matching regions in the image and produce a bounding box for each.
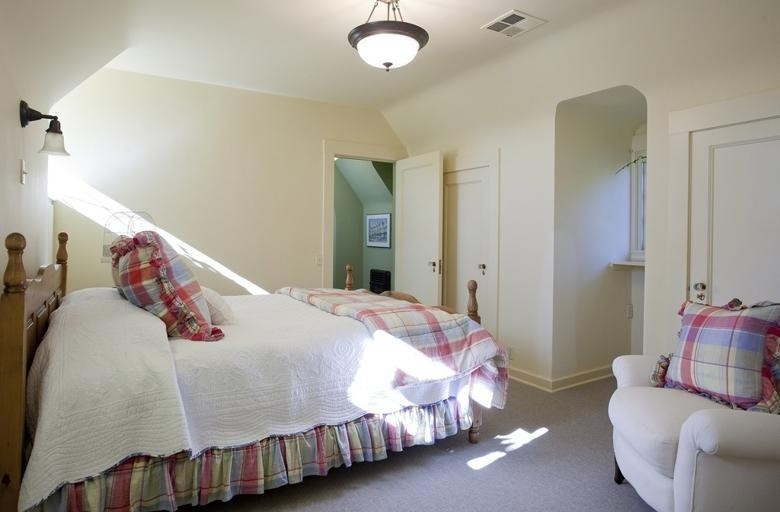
[1,223,490,512]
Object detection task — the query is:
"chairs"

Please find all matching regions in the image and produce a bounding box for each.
[607,353,779,512]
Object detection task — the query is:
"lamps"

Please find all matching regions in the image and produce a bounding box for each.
[19,99,71,158]
[347,0,432,72]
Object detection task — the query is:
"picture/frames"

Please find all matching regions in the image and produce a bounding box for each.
[364,212,392,249]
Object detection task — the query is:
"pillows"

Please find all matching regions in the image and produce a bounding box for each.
[650,296,780,415]
[110,228,236,341]
